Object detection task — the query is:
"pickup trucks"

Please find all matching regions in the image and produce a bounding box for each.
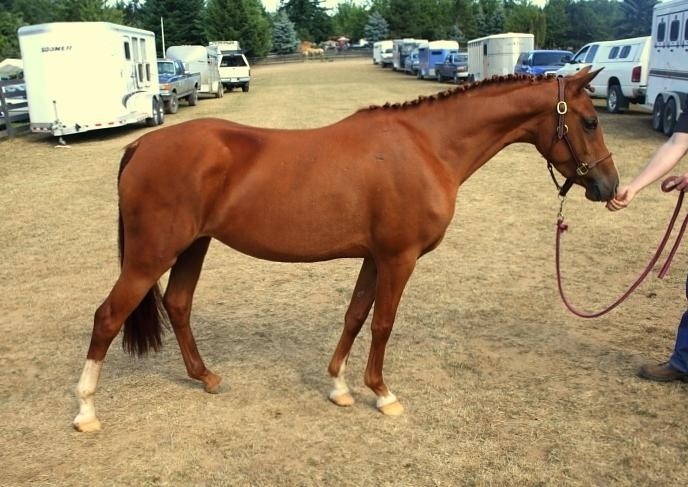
[157,57,201,113]
[380,46,393,66]
[434,51,469,83]
[404,48,420,73]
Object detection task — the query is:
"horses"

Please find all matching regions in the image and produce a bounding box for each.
[72,65,620,433]
[302,47,325,61]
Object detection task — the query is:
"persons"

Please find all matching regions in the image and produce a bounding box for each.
[606,93,688,382]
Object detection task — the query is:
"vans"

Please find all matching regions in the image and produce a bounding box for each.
[557,35,653,115]
[208,39,244,56]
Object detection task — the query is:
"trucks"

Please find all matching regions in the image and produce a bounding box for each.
[467,33,536,87]
[417,40,461,79]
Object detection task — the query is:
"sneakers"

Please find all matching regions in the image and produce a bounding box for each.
[641,362,688,382]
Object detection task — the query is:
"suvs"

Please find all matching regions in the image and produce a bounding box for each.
[513,48,576,79]
[217,54,250,94]
